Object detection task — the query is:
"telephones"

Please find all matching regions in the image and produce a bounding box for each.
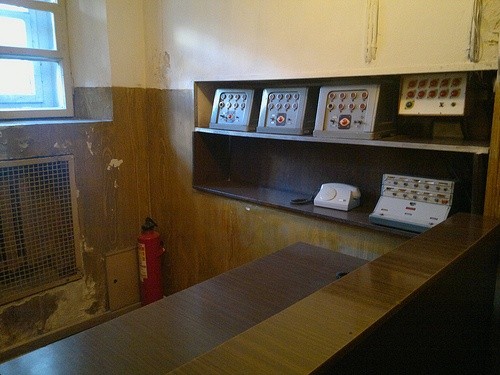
[314,182,362,212]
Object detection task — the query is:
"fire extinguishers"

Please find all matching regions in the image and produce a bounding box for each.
[138,216,166,307]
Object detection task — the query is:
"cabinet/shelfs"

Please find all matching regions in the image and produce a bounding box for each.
[190,126,490,239]
